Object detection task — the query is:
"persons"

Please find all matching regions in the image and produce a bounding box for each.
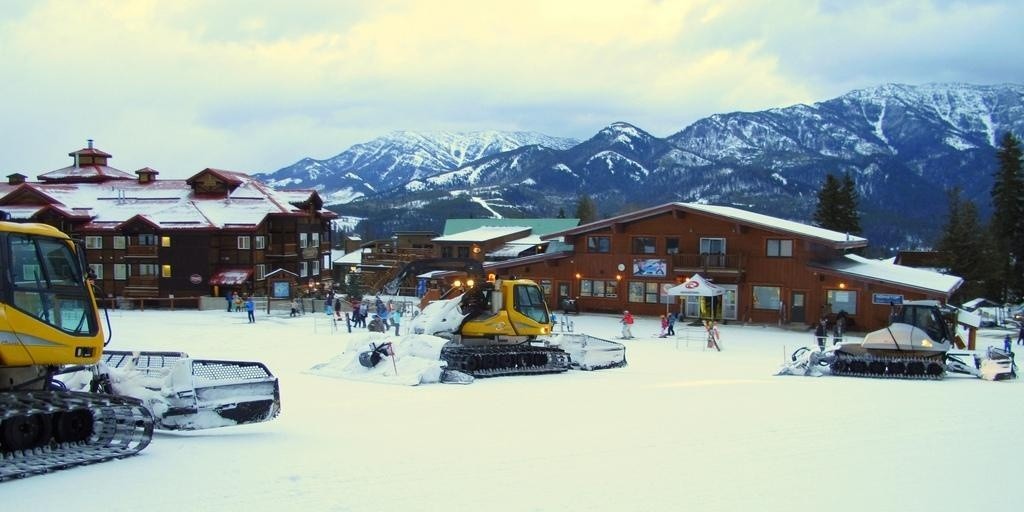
[833,318,843,346]
[815,319,828,353]
[706,321,720,349]
[1004,333,1011,354]
[1017,325,1024,346]
[549,309,557,331]
[618,311,634,339]
[225,288,402,337]
[658,314,668,338]
[368,340,394,365]
[665,312,676,336]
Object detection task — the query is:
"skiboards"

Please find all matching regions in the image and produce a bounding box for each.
[702,319,720,351]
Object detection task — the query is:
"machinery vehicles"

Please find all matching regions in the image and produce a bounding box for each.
[299,250,630,386]
[1,212,283,483]
[787,290,1015,381]
